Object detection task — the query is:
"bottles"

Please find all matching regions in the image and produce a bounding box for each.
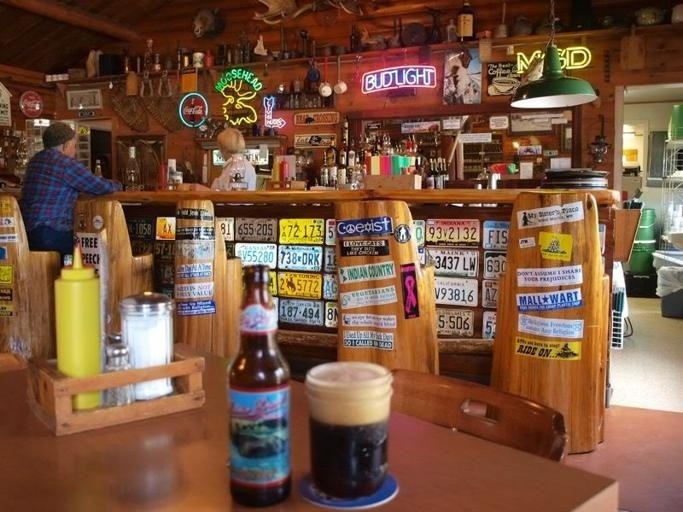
[117,291,174,402]
[446,0,475,43]
[630,187,644,209]
[123,146,141,193]
[227,264,291,507]
[279,157,289,189]
[271,156,280,190]
[277,76,331,108]
[120,41,252,73]
[93,159,102,177]
[305,132,502,192]
[621,190,631,209]
[231,173,248,191]
[103,345,132,408]
[53,242,103,411]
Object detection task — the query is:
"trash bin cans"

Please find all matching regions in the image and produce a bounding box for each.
[653,251,683,319]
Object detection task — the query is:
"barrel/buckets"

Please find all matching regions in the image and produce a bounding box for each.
[671,104,683,141]
[630,241,656,272]
[634,208,656,240]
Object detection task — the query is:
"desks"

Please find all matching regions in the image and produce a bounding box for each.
[0,347,623,511]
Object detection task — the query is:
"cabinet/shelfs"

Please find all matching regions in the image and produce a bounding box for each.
[390,123,502,182]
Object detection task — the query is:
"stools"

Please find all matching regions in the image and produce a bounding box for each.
[1,195,61,360]
[73,195,154,333]
[332,201,440,377]
[490,193,610,454]
[172,193,243,360]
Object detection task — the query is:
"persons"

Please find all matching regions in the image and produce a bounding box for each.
[190,128,256,192]
[19,122,122,267]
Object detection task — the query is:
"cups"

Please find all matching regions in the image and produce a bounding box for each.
[169,170,184,190]
[304,360,394,501]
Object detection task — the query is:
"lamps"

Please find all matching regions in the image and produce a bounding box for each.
[507,1,611,165]
